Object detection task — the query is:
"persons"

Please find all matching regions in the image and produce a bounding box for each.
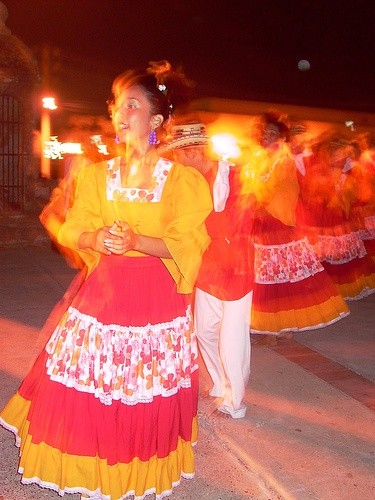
[168,118,258,420]
[240,109,300,339]
[30,61,213,500]
